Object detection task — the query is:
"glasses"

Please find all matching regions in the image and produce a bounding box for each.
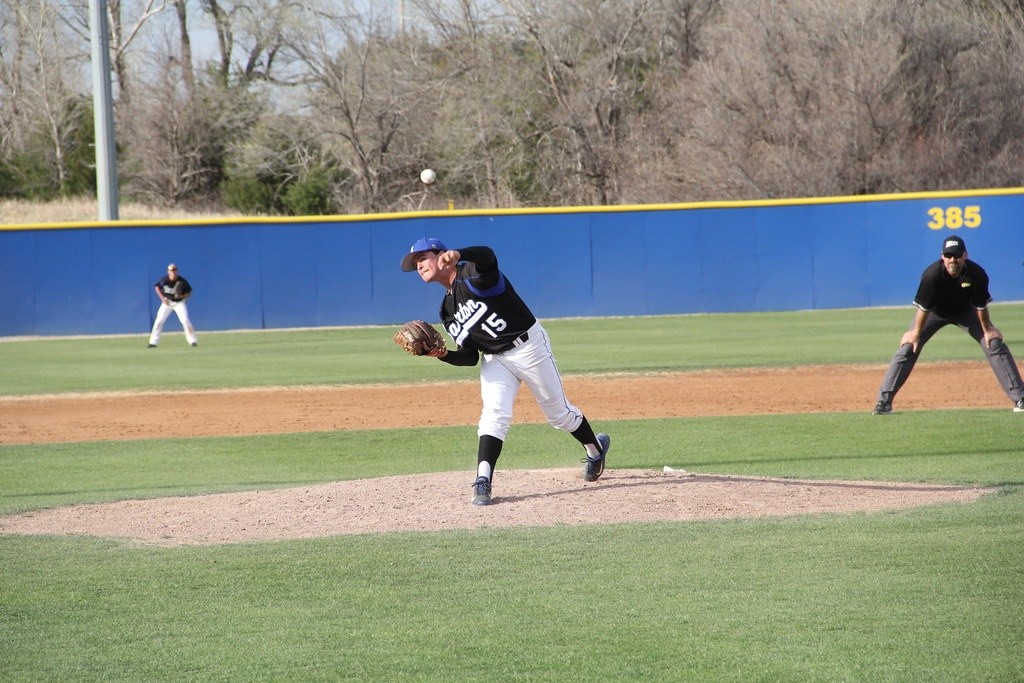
[944,253,962,259]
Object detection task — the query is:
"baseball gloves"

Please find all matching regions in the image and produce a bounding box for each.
[173,281,184,299]
[393,320,447,357]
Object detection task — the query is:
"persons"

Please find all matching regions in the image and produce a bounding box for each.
[393,236,610,505]
[147,263,199,347]
[871,235,1024,416]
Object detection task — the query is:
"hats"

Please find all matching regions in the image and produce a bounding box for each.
[167,264,177,271]
[401,237,447,272]
[941,235,965,253]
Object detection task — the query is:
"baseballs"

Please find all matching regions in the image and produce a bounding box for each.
[420,169,436,185]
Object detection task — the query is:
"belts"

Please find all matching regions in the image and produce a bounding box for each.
[501,333,528,352]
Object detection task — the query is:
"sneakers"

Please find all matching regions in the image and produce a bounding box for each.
[1014,400,1024,412]
[471,477,491,506]
[872,403,893,415]
[581,433,610,482]
